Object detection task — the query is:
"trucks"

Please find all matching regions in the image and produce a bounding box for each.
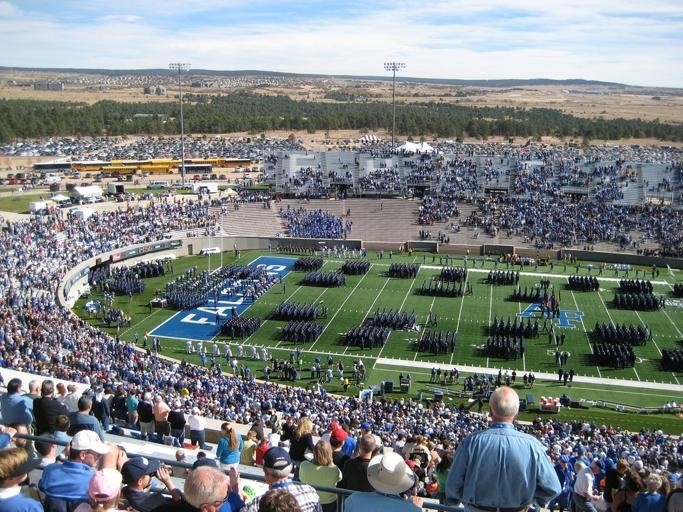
[105,182,125,196]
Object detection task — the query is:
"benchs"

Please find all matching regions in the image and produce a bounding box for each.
[160,152,683,261]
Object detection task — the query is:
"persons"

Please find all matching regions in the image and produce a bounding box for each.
[0,133,681,512]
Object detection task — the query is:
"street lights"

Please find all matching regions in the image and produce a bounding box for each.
[166,62,194,185]
[381,61,406,148]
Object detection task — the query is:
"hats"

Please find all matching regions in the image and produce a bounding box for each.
[332,428,348,443]
[262,448,292,469]
[0,454,42,482]
[367,451,416,495]
[70,429,111,455]
[122,457,161,484]
[358,422,371,429]
[596,459,606,473]
[89,468,123,502]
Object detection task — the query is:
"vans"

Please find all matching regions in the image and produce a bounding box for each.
[146,181,169,190]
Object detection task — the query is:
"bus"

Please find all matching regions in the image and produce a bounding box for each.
[176,164,214,174]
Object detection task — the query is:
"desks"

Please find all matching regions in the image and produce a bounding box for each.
[187,413,250,445]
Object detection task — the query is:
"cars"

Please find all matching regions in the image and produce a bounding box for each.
[0,136,298,163]
[191,174,227,181]
[0,169,149,188]
[234,165,276,180]
